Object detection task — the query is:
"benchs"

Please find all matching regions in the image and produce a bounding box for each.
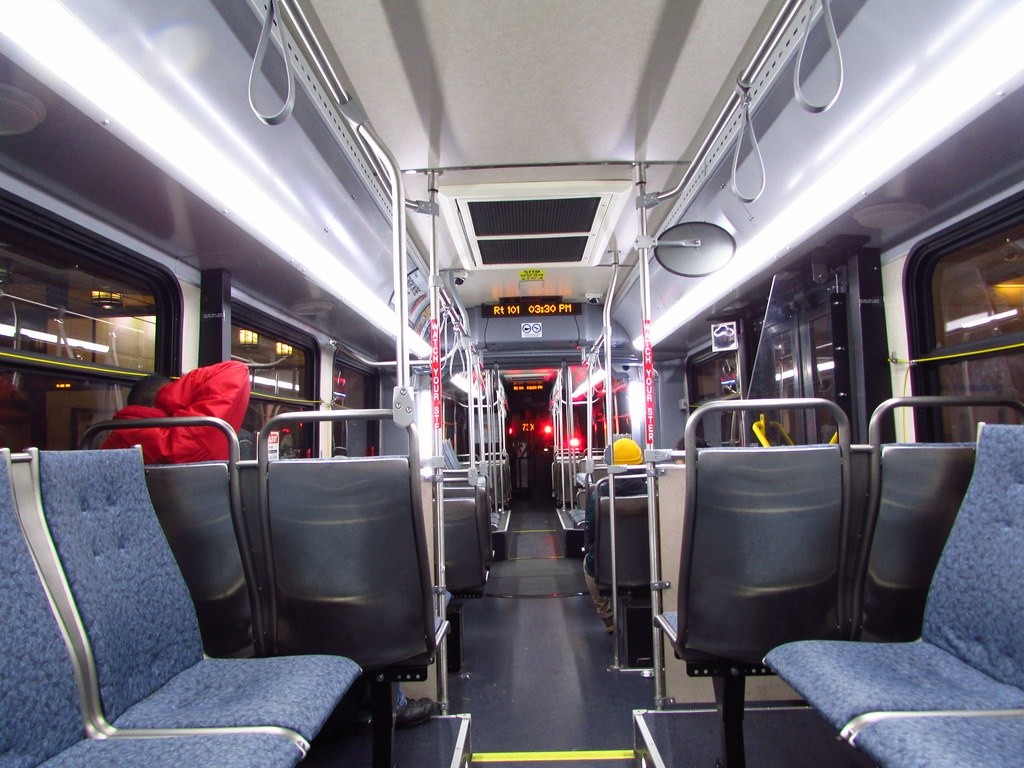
[0,398,1024,768]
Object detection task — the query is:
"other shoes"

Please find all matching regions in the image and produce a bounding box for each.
[603,616,614,632]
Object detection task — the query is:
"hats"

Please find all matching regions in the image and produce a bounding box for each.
[614,437,643,465]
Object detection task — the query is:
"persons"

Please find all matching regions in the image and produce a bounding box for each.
[99,360,252,464]
[672,436,708,464]
[582,438,647,633]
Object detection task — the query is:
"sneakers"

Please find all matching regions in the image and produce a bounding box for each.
[352,697,435,732]
